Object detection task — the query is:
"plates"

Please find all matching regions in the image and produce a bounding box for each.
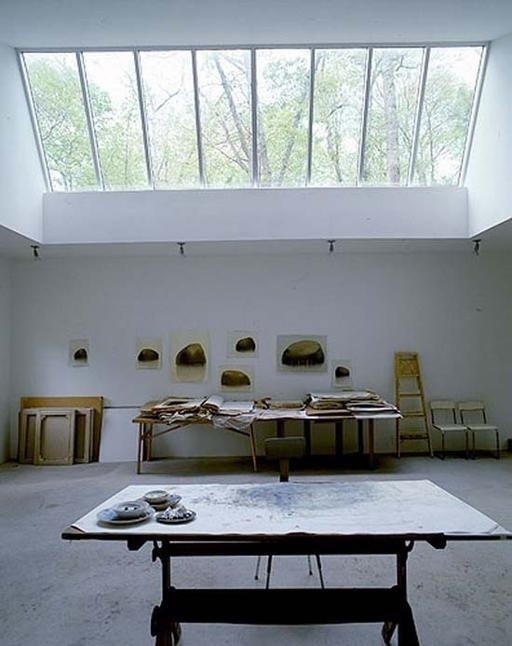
[156,510,196,524]
[96,507,155,525]
[136,495,182,509]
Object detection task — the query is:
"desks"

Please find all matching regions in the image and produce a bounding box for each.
[255,399,402,471]
[60,484,512,646]
[132,396,266,472]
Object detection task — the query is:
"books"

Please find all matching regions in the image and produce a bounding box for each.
[149,394,256,416]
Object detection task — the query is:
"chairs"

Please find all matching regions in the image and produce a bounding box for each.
[456,399,500,460]
[430,399,469,460]
[253,436,326,589]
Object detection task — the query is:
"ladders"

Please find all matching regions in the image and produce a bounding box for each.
[395,351,433,458]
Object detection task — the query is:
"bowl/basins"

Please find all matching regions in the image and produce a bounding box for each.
[143,490,170,503]
[115,501,145,517]
